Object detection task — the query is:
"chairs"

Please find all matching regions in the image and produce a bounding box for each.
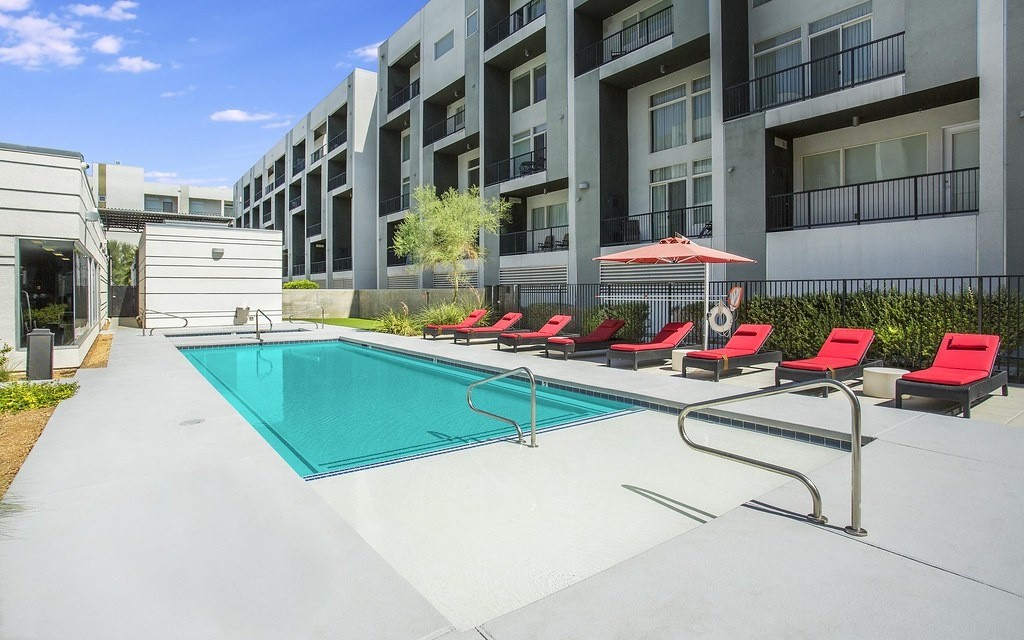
[498,314,580,353]
[454,312,533,346]
[423,309,488,341]
[518,157,544,177]
[555,234,569,251]
[606,321,704,371]
[545,319,635,361]
[682,323,782,383]
[895,332,1008,418]
[538,236,554,252]
[775,327,883,398]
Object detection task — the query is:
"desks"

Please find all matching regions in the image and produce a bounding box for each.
[862,367,910,399]
[672,350,702,371]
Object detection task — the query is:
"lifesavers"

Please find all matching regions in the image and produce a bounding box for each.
[708,306,733,332]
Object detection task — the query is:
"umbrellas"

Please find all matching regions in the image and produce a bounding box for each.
[594,237,757,355]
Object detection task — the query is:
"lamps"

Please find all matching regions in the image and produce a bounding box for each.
[525,49,530,57]
[212,248,224,253]
[85,211,98,221]
[853,117,859,127]
